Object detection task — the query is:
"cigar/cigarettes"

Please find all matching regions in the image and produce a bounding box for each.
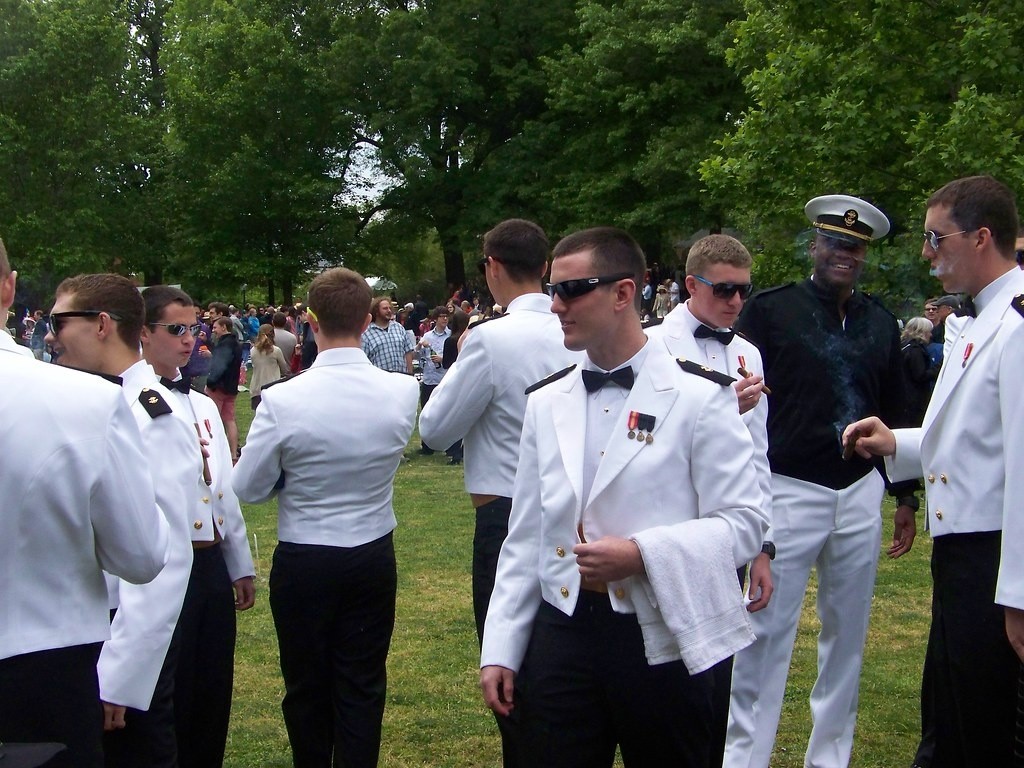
[194,423,212,487]
[842,427,862,461]
[737,368,772,395]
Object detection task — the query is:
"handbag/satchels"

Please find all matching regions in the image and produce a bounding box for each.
[239,367,247,385]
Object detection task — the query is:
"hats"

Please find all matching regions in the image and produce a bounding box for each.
[229,305,234,308]
[404,303,414,308]
[664,279,673,284]
[656,285,668,293]
[804,195,890,245]
[931,295,958,308]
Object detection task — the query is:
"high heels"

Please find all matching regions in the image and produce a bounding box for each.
[447,458,461,465]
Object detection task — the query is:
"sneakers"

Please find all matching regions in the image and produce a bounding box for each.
[400,455,410,463]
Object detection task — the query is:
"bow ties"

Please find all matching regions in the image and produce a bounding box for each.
[160,376,191,394]
[582,365,635,393]
[953,295,977,319]
[694,324,735,345]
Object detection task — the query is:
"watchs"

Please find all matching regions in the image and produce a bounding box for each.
[895,496,919,512]
[761,543,776,560]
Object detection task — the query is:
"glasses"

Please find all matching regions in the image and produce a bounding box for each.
[691,275,754,300]
[923,231,971,252]
[438,315,451,319]
[546,273,635,301]
[476,257,507,276]
[924,308,938,310]
[148,323,201,337]
[49,311,124,337]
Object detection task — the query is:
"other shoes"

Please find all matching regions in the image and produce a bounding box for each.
[418,450,432,455]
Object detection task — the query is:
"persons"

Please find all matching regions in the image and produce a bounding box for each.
[231,268,420,768]
[139,285,257,768]
[22,309,47,361]
[0,238,173,768]
[842,175,1024,768]
[180,302,318,467]
[643,262,680,318]
[44,274,195,768]
[419,219,586,652]
[480,227,772,768]
[901,295,960,386]
[644,235,774,613]
[721,194,917,768]
[363,281,507,465]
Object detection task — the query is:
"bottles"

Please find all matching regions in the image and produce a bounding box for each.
[429,345,442,369]
